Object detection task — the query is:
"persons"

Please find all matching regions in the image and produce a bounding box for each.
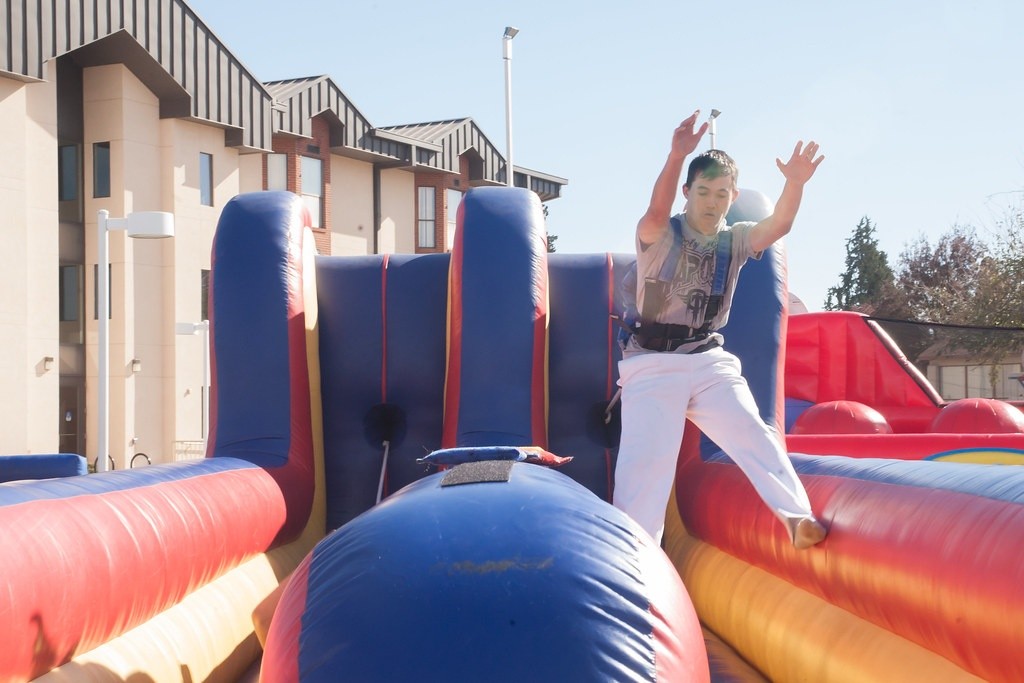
[611,110,829,548]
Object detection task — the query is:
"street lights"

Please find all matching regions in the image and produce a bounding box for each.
[708,108,722,149]
[502,27,522,188]
